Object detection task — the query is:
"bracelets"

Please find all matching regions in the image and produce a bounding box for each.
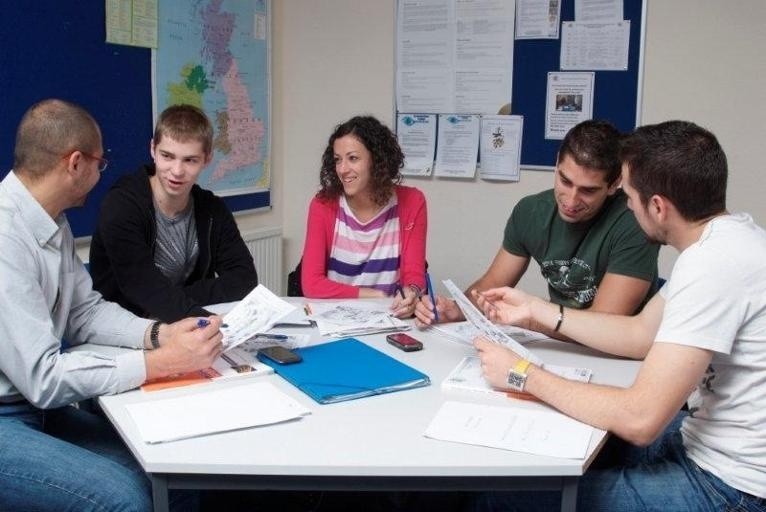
[150,320,164,349]
[410,284,422,298]
[553,303,564,331]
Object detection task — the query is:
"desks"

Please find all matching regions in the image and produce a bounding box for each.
[97,296,644,512]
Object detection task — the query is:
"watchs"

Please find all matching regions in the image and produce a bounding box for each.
[505,358,531,392]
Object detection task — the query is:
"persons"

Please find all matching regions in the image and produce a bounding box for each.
[0,96,227,512]
[471,120,766,512]
[88,103,259,326]
[414,119,662,344]
[299,114,429,320]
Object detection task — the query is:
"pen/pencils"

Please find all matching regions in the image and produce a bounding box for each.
[396,281,407,300]
[304,306,314,328]
[198,320,228,329]
[425,272,440,326]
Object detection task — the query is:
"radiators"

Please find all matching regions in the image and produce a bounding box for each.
[244,226,285,296]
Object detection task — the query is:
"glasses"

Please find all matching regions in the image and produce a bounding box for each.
[61,148,108,173]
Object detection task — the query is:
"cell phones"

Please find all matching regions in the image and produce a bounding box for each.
[387,332,423,352]
[257,344,301,365]
